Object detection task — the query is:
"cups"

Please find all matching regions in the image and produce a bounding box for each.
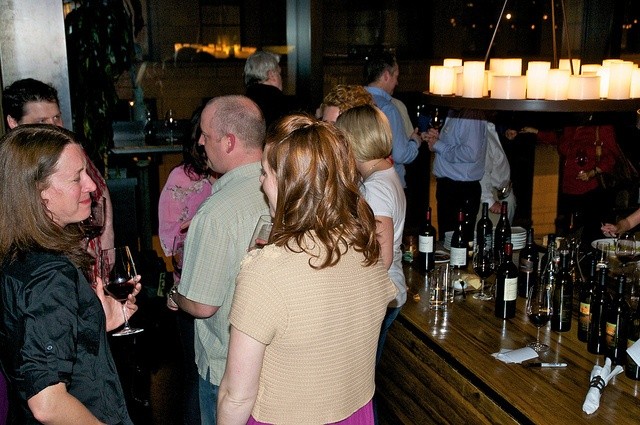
[429,264,455,310]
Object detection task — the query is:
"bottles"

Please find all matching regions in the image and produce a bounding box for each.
[517,227,539,299]
[542,233,556,284]
[449,208,469,270]
[494,201,512,261]
[576,259,601,343]
[494,243,518,320]
[551,251,573,333]
[587,268,612,355]
[604,273,630,366]
[416,206,437,276]
[625,294,640,381]
[476,202,493,258]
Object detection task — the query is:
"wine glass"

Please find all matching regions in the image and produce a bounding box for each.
[164,109,179,144]
[491,177,512,201]
[471,251,494,301]
[99,244,144,339]
[248,214,274,251]
[574,147,589,174]
[596,241,611,264]
[78,201,104,253]
[615,231,635,270]
[172,235,184,279]
[525,285,554,355]
[429,105,444,131]
[413,105,426,134]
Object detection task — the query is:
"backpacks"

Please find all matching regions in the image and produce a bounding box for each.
[598,142,637,193]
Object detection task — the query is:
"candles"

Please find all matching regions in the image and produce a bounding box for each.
[429,58,639,100]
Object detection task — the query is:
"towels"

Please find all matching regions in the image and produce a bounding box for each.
[582,357,624,414]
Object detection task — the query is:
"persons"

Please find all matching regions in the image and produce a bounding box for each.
[216,111,401,425]
[169,94,273,425]
[391,97,415,141]
[361,47,423,243]
[423,36,488,242]
[0,371,10,425]
[0,78,116,295]
[0,123,143,425]
[244,50,305,134]
[159,102,223,312]
[519,125,621,247]
[315,85,378,124]
[474,122,517,227]
[335,105,407,424]
[601,188,640,240]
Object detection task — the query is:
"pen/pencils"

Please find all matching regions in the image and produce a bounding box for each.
[528,363,567,367]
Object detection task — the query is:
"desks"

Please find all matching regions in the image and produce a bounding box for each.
[111,123,184,248]
[375,237,639,425]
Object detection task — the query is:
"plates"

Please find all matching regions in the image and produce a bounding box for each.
[443,230,454,252]
[591,238,640,258]
[434,249,451,264]
[511,226,527,250]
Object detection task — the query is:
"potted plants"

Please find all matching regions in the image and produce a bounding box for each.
[64,0,139,257]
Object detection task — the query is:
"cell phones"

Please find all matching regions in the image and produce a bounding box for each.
[433,254,449,264]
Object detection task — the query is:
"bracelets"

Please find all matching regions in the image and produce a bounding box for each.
[592,167,599,176]
[408,139,417,145]
[169,286,177,297]
